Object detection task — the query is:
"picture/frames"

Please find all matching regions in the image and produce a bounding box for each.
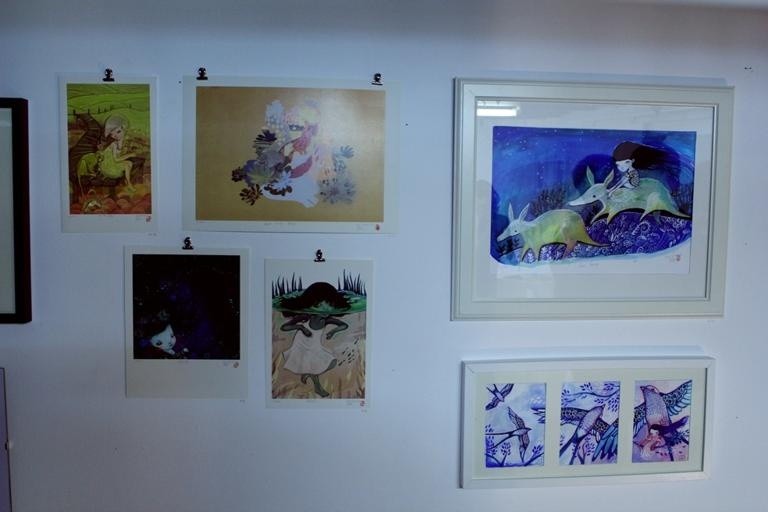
[0,98,32,324]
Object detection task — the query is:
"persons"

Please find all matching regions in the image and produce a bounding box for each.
[142,315,191,359]
[604,140,672,200]
[274,281,354,396]
[93,113,138,192]
